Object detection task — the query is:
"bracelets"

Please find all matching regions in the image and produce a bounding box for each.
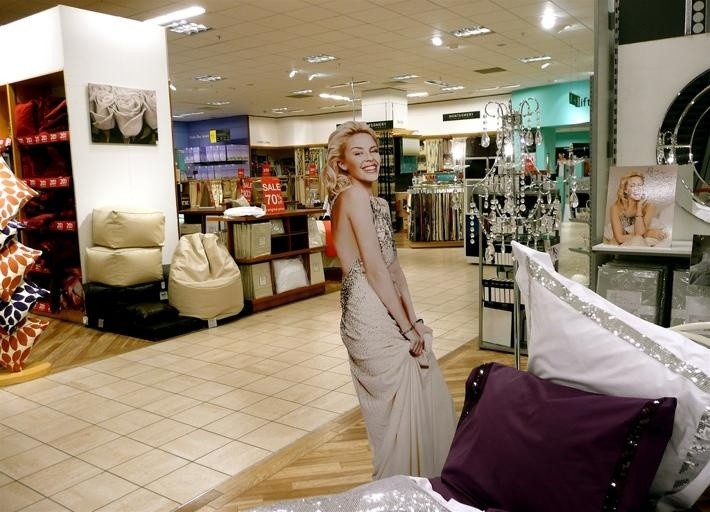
[635,214,644,217]
[411,318,425,331]
[641,234,645,239]
[400,326,415,336]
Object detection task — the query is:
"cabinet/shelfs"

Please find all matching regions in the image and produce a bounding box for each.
[477,190,559,357]
[14,131,82,316]
[204,209,327,313]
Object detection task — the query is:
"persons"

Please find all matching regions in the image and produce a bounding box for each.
[608,170,669,248]
[319,121,456,480]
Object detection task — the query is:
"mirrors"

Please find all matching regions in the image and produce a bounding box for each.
[655,68,710,226]
